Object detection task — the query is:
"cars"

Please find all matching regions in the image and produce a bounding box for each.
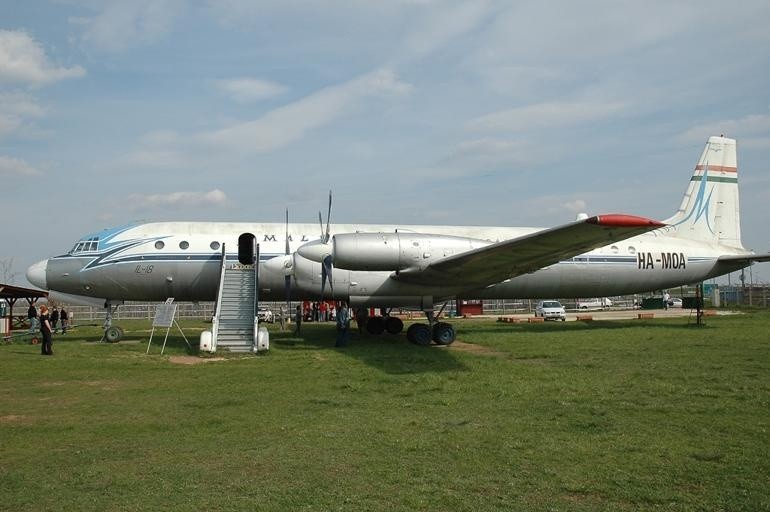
[274,308,296,324]
[634,297,683,309]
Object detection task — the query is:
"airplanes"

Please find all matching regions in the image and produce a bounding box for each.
[23,133,770,359]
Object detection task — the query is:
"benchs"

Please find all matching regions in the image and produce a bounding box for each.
[577,316,592,321]
[498,317,520,323]
[638,313,654,318]
[528,317,545,322]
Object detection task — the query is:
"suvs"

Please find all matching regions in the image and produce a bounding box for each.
[576,297,612,309]
[535,299,567,322]
[257,306,273,323]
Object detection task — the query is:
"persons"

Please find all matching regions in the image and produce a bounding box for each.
[334,306,350,347]
[303,301,337,322]
[28,301,38,332]
[263,308,272,322]
[293,305,305,337]
[61,307,68,333]
[40,305,55,355]
[51,306,60,334]
[663,290,670,311]
